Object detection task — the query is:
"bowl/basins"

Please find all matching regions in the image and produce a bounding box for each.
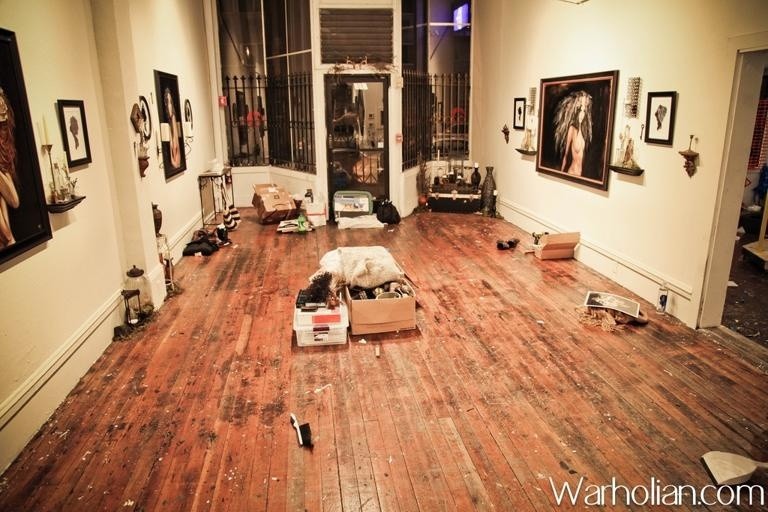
[376,291,402,300]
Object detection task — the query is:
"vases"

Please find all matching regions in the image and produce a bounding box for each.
[152,204,162,238]
[478,166,500,218]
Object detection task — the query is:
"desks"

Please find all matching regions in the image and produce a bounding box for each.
[156,234,175,291]
[197,164,235,228]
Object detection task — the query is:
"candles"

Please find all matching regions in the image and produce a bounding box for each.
[159,122,170,143]
[42,113,50,146]
[182,121,193,137]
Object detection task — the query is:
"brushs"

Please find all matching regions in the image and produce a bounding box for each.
[290,413,312,448]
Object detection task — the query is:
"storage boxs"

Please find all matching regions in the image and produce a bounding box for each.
[251,190,295,225]
[393,259,419,290]
[341,276,418,336]
[532,229,584,261]
[291,298,351,347]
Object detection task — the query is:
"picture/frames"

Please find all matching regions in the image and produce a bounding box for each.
[56,98,93,168]
[535,68,620,191]
[153,69,188,180]
[513,97,526,130]
[0,27,55,267]
[644,89,677,145]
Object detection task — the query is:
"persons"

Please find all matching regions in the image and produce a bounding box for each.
[553,90,594,176]
[333,109,358,135]
[0,88,20,247]
[162,88,182,169]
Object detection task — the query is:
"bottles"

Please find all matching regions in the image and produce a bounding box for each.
[152,205,163,237]
[471,168,481,189]
[123,264,152,317]
[481,167,496,213]
[297,212,306,234]
[656,286,668,315]
